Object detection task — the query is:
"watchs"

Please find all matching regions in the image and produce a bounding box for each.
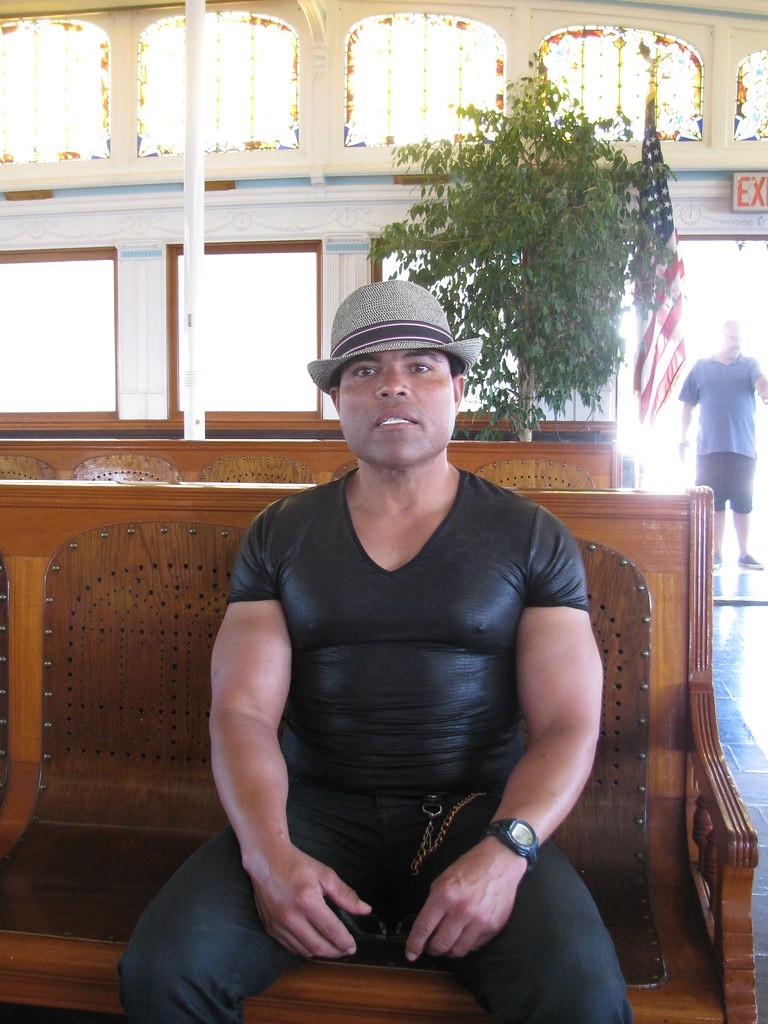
[477,817,539,874]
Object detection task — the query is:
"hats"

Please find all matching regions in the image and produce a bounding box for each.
[308,280,484,394]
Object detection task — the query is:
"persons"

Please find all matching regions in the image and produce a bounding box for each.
[116,281,639,1024]
[676,319,768,569]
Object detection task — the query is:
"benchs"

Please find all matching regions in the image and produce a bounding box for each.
[0,472,759,1024]
[0,411,624,494]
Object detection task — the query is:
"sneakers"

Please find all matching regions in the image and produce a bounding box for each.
[713,555,722,570]
[738,554,763,571]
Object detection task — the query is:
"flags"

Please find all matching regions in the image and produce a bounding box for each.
[632,100,686,426]
